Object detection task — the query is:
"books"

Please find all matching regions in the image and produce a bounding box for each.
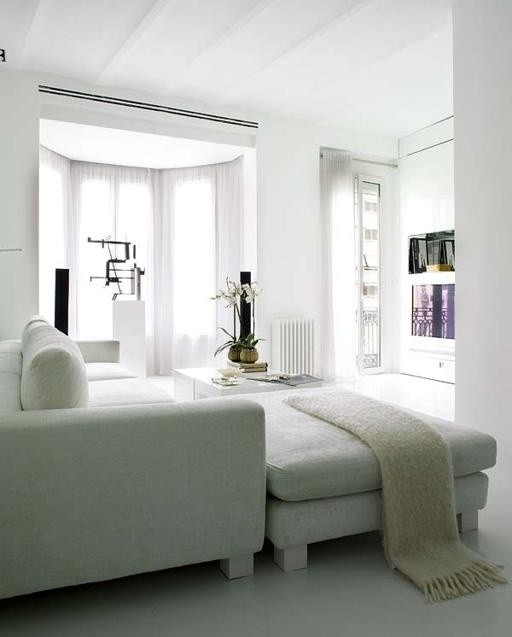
[408,231,455,274]
[279,373,323,387]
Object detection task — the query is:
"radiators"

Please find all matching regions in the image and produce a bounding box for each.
[272,318,315,378]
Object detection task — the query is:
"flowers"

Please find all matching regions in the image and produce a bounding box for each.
[239,280,267,350]
[209,275,244,358]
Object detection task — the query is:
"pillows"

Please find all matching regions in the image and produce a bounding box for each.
[18,314,90,413]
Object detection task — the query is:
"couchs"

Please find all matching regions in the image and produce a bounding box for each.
[204,385,498,574]
[1,340,269,604]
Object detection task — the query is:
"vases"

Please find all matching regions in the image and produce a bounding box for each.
[239,349,259,364]
[227,347,244,363]
